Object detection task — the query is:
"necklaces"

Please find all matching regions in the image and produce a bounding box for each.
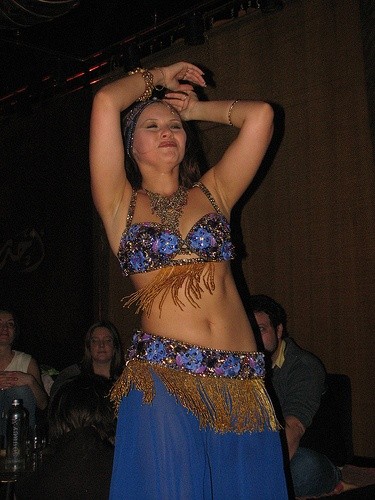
[145,185,187,237]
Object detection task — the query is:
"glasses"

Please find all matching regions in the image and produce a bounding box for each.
[0,322,13,328]
[88,336,111,344]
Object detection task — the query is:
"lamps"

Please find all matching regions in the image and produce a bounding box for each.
[123,45,142,72]
[185,13,207,46]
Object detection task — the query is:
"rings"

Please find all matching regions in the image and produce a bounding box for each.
[185,96,186,100]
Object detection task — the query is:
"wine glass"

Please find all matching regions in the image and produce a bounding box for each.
[29,424,45,469]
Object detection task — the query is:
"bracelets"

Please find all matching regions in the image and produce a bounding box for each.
[128,67,153,101]
[154,69,165,91]
[228,99,239,126]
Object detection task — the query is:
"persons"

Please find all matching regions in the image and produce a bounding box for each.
[90,61,287,500]
[50,321,124,396]
[0,310,48,450]
[248,294,339,496]
[5,380,116,500]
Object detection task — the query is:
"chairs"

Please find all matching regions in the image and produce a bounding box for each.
[306,373,354,465]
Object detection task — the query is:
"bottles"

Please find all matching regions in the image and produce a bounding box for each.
[3,399,27,471]
